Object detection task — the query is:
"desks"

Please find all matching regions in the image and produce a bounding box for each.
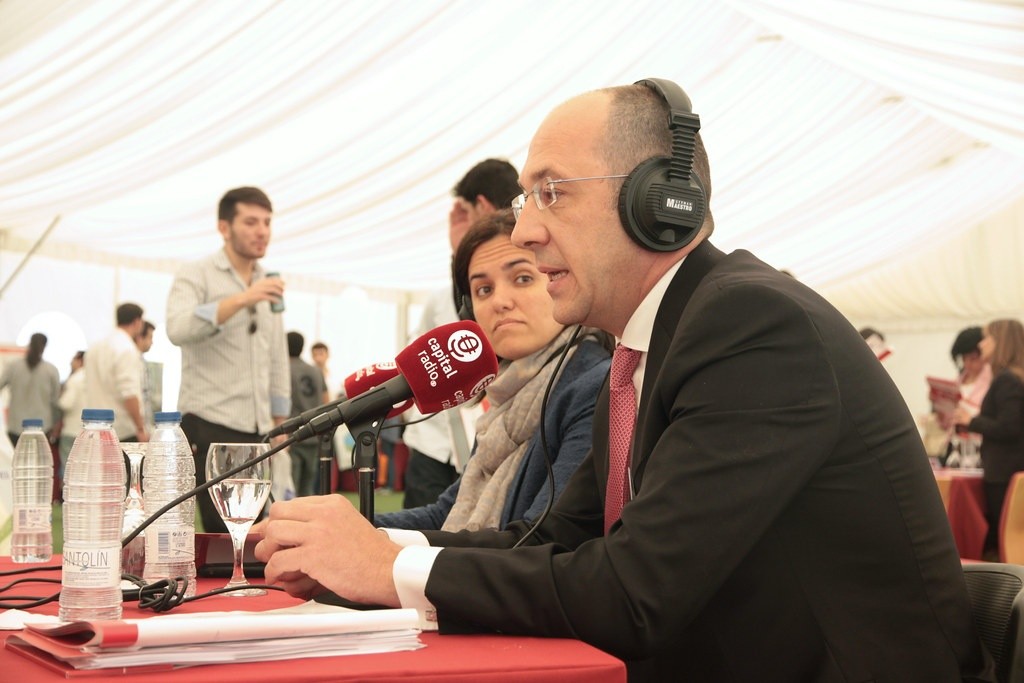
[932,467,991,560]
[0,548,628,682]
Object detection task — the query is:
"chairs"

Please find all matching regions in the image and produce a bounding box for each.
[962,563,1024,683]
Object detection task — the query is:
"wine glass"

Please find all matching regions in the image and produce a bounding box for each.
[204,443,275,598]
[118,441,147,539]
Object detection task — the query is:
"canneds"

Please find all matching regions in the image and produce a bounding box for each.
[266,272,284,313]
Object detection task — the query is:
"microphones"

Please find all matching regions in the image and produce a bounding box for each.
[268,319,500,441]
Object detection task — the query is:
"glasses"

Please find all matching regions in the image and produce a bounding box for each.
[510,175,630,221]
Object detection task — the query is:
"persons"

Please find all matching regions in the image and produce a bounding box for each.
[0,303,156,505]
[449,133,544,323]
[254,86,980,683]
[286,331,400,498]
[929,319,1024,563]
[166,186,291,534]
[375,208,612,522]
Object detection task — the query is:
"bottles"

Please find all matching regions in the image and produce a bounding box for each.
[9,419,53,564]
[58,409,127,622]
[139,411,198,600]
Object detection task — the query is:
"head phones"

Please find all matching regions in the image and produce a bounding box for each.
[617,77,707,253]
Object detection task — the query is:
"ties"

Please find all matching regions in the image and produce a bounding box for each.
[604,345,643,536]
